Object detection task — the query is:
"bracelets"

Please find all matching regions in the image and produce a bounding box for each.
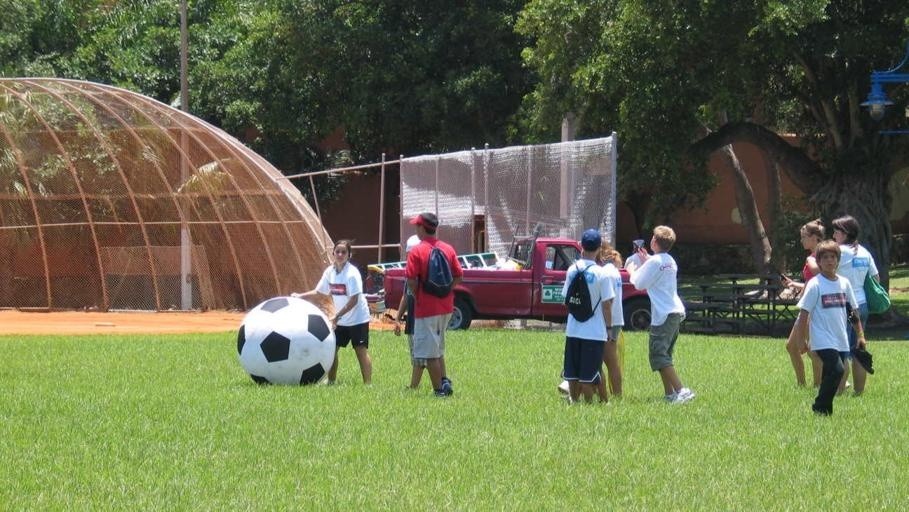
[856,332,865,336]
[607,326,613,329]
[336,312,342,319]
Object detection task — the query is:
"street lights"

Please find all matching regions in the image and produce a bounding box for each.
[859,68,909,120]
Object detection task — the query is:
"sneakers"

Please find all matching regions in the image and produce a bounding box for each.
[665,387,695,403]
[852,344,875,374]
[434,377,454,397]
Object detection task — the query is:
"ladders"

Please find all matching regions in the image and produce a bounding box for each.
[367,252,500,274]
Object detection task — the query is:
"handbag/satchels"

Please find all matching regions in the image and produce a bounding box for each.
[864,274,891,314]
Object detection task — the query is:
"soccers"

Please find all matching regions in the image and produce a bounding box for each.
[237,297,337,387]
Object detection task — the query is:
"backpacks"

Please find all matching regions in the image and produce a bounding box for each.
[423,243,455,297]
[565,263,601,322]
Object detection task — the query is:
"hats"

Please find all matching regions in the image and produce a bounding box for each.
[409,215,438,230]
[581,228,601,252]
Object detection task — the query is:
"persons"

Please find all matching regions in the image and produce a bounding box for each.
[393,234,428,388]
[832,214,880,394]
[781,218,824,388]
[626,225,695,404]
[561,227,616,403]
[795,238,866,414]
[291,240,373,387]
[404,212,464,399]
[596,240,624,402]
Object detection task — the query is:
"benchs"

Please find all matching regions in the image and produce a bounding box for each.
[678,273,806,336]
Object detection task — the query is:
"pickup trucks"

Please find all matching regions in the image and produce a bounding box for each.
[382,236,653,333]
